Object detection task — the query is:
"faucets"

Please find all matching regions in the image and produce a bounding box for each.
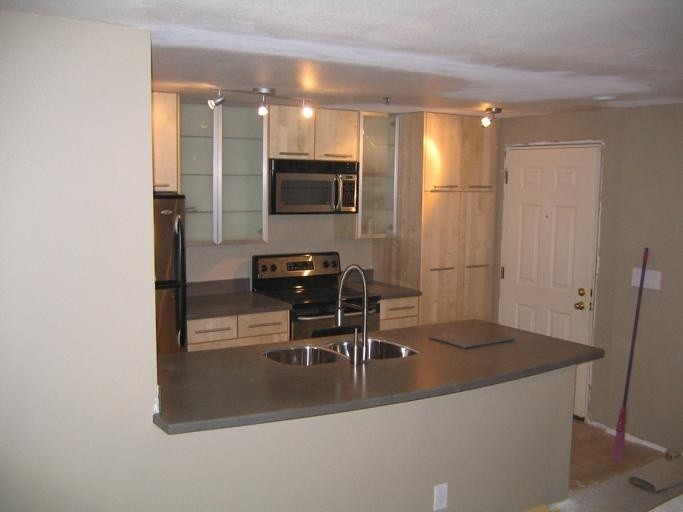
[334,264,369,361]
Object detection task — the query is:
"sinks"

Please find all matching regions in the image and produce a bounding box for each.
[260,345,349,368]
[328,338,420,360]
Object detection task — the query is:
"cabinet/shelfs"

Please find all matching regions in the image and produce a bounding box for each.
[152,91,180,193]
[374,115,496,328]
[375,296,419,331]
[178,98,268,246]
[268,103,360,162]
[185,310,290,353]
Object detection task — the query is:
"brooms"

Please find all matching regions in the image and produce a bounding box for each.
[610,248,649,461]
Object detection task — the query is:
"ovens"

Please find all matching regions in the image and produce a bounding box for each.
[289,300,381,342]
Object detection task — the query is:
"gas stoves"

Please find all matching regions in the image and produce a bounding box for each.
[255,283,380,309]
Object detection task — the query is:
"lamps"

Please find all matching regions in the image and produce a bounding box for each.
[207,87,275,116]
[335,114,399,241]
[480,107,502,127]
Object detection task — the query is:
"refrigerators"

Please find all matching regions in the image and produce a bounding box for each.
[153,192,190,356]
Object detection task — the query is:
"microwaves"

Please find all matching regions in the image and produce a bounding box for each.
[268,157,360,216]
